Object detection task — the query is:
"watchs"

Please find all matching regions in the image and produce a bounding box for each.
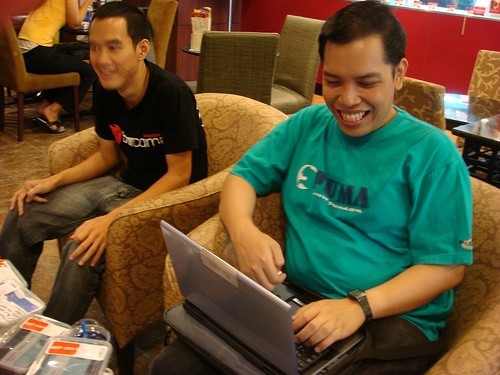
[348,288,373,323]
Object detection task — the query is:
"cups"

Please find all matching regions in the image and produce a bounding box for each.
[64,318,111,341]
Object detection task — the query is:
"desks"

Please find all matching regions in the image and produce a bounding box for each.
[60,24,89,36]
[444,94,500,189]
[182,46,200,57]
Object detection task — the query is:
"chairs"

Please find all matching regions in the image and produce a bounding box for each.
[185,31,279,106]
[270,15,326,115]
[0,7,80,142]
[393,77,447,132]
[467,50,500,103]
[146,0,179,68]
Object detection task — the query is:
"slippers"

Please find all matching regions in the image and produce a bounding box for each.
[35,114,66,133]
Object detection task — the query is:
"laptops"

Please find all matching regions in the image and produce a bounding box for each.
[161,220,365,375]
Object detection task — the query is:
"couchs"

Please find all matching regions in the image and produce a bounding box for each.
[163,174,500,375]
[47,93,290,375]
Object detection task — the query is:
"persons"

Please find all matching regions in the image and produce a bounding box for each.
[216,0,473,374]
[0,1,208,325]
